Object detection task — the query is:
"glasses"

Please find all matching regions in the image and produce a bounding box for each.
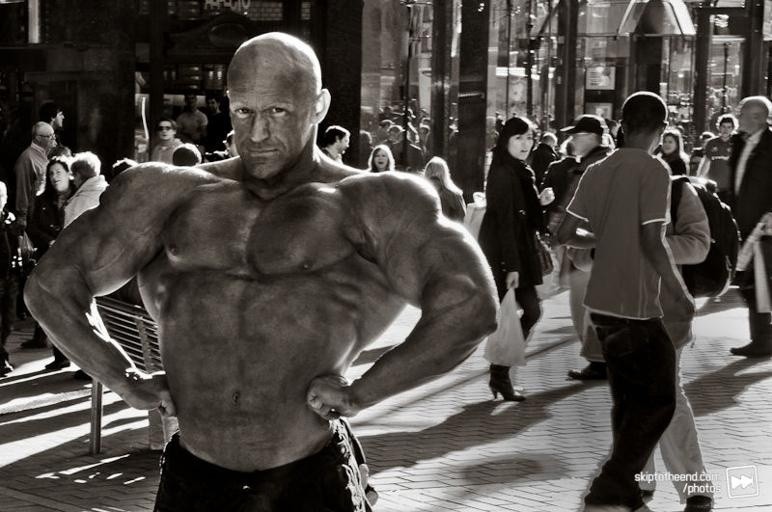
[40,132,55,138]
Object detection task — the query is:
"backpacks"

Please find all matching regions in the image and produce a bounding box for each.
[676,178,744,296]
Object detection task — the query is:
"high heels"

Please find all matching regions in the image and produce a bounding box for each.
[486,374,529,405]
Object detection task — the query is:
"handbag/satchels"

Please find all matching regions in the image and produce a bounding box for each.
[535,231,556,277]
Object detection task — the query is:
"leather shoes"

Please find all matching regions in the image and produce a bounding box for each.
[727,340,771,357]
[567,359,609,381]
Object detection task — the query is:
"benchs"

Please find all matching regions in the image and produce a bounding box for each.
[89,296,162,454]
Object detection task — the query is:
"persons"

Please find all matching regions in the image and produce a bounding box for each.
[1,32,771,512]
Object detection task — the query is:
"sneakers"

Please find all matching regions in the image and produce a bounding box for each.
[18,337,48,349]
[44,355,70,370]
[576,478,714,511]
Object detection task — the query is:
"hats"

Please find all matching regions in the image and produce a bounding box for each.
[555,113,608,137]
